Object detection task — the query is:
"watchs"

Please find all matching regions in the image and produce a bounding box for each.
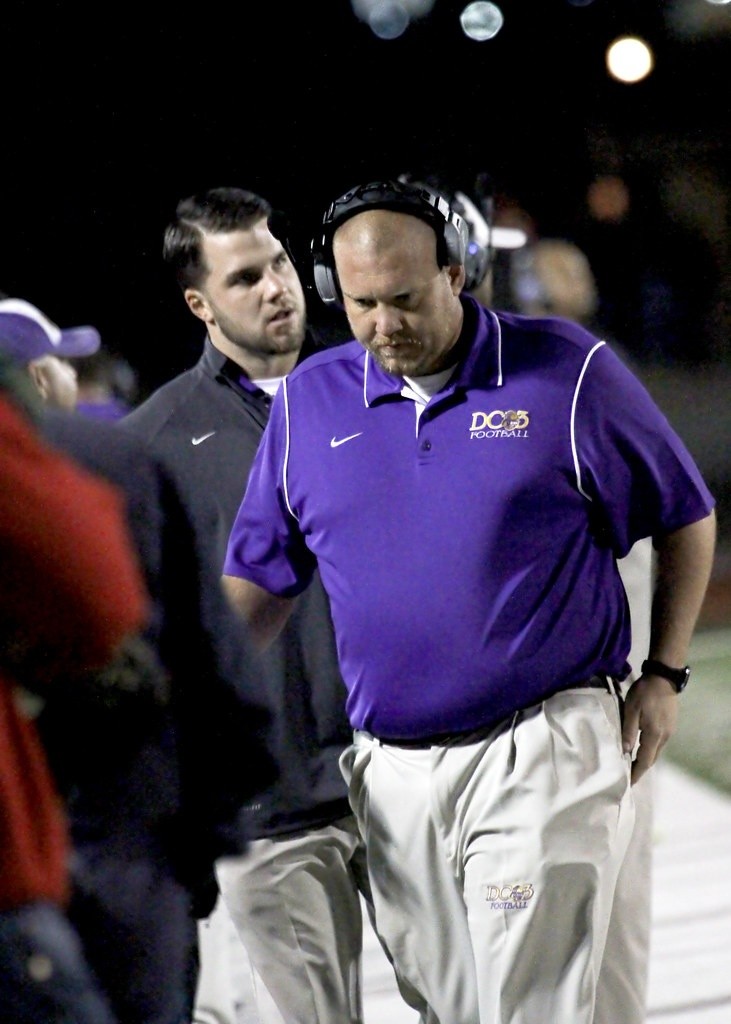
[642,659,690,693]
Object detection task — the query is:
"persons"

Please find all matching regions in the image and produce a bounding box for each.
[223,174,715,1024]
[114,188,367,1024]
[0,298,221,1023]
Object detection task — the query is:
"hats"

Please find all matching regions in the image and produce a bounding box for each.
[2,297,102,363]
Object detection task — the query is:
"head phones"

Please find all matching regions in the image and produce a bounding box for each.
[309,177,488,313]
